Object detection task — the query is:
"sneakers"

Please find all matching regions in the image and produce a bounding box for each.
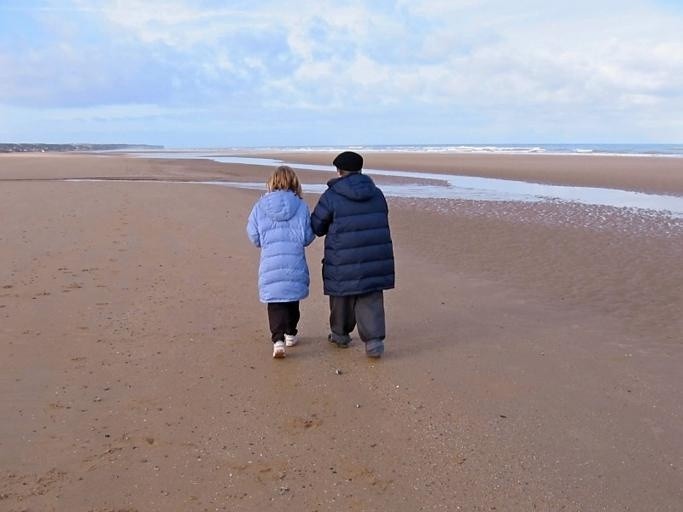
[283,332,299,347]
[366,344,385,359]
[272,339,288,359]
[327,332,351,349]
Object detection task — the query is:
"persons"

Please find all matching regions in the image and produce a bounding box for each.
[310,151,394,358]
[245,166,316,359]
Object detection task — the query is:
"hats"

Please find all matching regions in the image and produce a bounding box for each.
[332,150,365,173]
